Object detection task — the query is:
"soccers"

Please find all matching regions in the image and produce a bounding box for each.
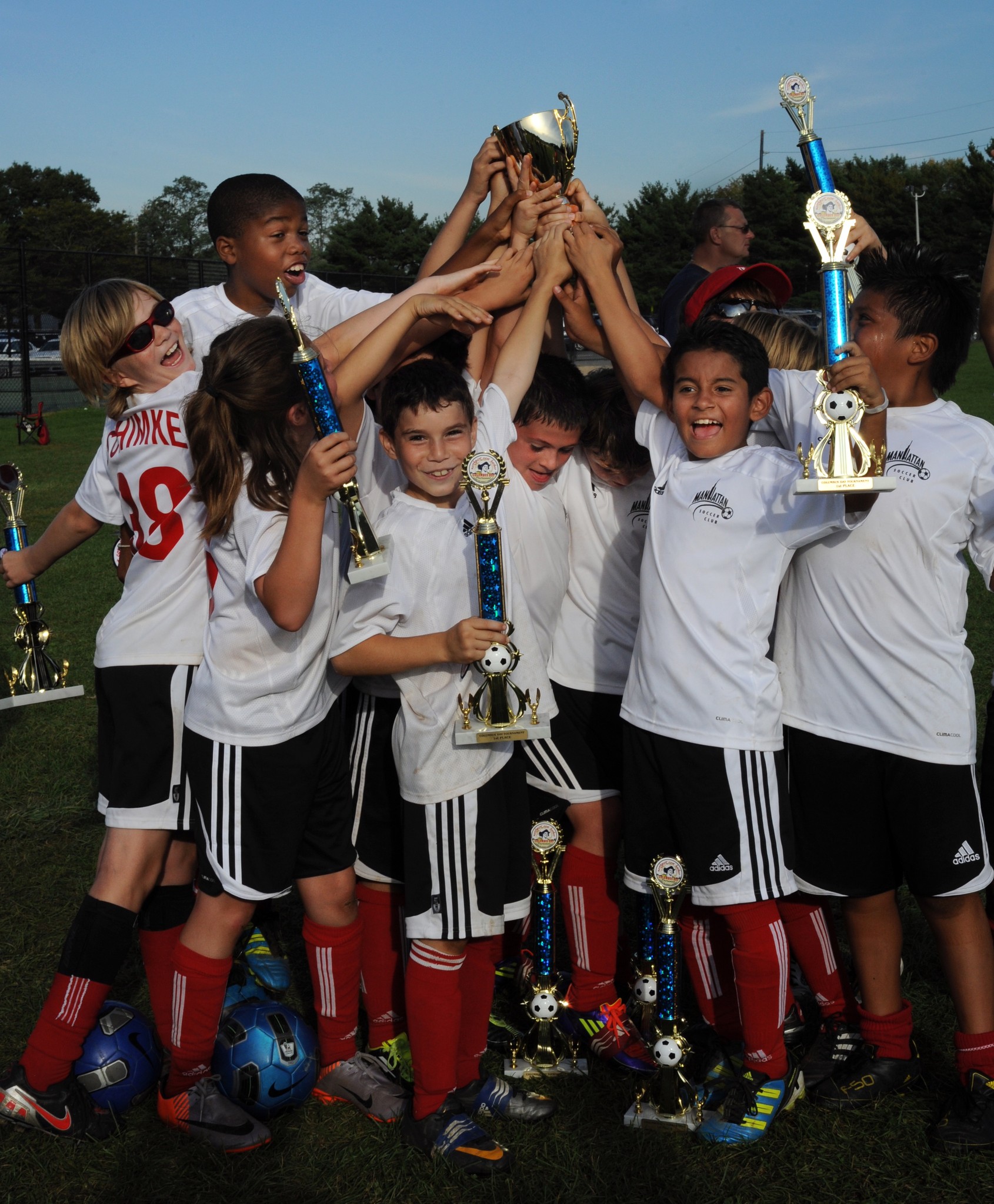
[210,999,319,1122]
[69,1001,166,1108]
[222,961,268,1008]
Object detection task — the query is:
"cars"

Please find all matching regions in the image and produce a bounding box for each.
[0,338,40,379]
[28,338,67,376]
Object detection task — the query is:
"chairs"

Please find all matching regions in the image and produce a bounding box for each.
[15,401,49,445]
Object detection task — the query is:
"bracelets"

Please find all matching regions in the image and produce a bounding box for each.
[862,387,889,414]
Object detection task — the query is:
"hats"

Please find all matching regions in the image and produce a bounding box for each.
[685,262,792,327]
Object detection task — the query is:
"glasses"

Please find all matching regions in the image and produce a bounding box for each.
[710,298,780,321]
[106,300,175,369]
[717,224,754,234]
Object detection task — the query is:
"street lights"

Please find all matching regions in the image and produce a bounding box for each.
[910,184,929,261]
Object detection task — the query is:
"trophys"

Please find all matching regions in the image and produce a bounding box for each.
[454,450,551,745]
[491,93,579,221]
[503,819,589,1076]
[791,189,897,493]
[274,277,395,585]
[0,462,85,709]
[623,855,704,1131]
[779,74,865,307]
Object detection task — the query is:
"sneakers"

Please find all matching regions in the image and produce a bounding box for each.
[702,1064,810,1148]
[360,1032,422,1087]
[314,1052,414,1125]
[953,1065,994,1124]
[805,1018,864,1081]
[494,950,530,982]
[814,1040,931,1108]
[560,996,665,1071]
[401,1090,510,1175]
[783,1011,825,1057]
[238,927,303,995]
[488,1004,540,1045]
[158,1067,271,1153]
[0,1064,91,1141]
[458,1071,551,1123]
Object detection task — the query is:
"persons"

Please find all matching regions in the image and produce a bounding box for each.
[659,202,756,342]
[327,222,572,1173]
[0,137,994,1166]
[564,221,887,1146]
